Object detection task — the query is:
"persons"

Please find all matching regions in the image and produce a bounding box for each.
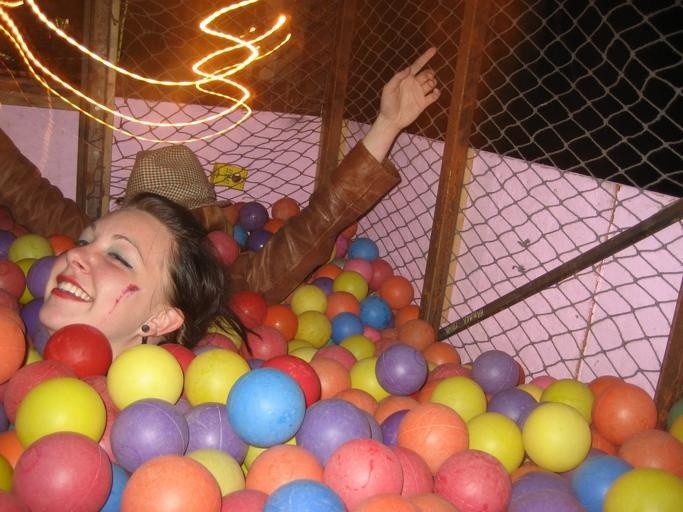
[39,192,225,366]
[0,44,443,308]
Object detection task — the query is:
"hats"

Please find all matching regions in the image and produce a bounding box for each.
[117,145,230,211]
